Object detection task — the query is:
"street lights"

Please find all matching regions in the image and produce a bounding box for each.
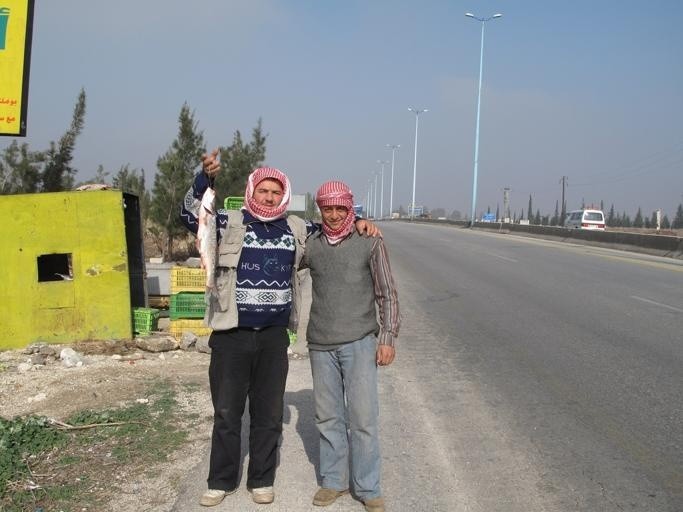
[407,105,429,224]
[385,143,400,220]
[462,12,503,225]
[362,160,391,220]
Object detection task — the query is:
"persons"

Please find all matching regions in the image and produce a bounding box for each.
[297,181,402,511]
[180,148,383,505]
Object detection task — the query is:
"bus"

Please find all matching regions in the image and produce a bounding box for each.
[352,204,362,218]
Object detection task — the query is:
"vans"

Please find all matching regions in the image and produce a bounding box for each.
[562,209,607,232]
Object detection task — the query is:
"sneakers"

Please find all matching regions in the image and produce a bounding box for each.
[199,488,237,506]
[248,486,274,503]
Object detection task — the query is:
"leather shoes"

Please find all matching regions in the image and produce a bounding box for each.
[313,487,351,506]
[364,497,385,512]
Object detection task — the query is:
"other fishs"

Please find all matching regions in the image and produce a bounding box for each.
[196,186,224,326]
